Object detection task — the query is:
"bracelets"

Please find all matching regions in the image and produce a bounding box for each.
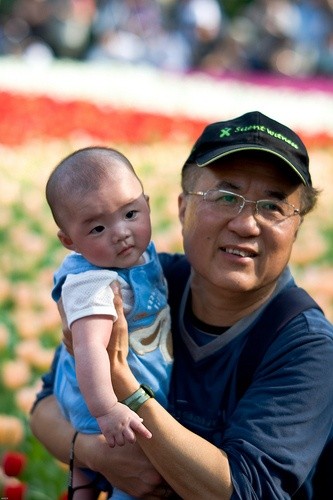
[122,384,154,411]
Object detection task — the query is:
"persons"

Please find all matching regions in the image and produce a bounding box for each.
[31,113,333,499]
[46,146,175,500]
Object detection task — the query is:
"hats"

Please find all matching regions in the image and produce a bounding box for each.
[182,111,312,189]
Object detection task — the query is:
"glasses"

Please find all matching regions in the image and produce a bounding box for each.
[187,189,301,221]
